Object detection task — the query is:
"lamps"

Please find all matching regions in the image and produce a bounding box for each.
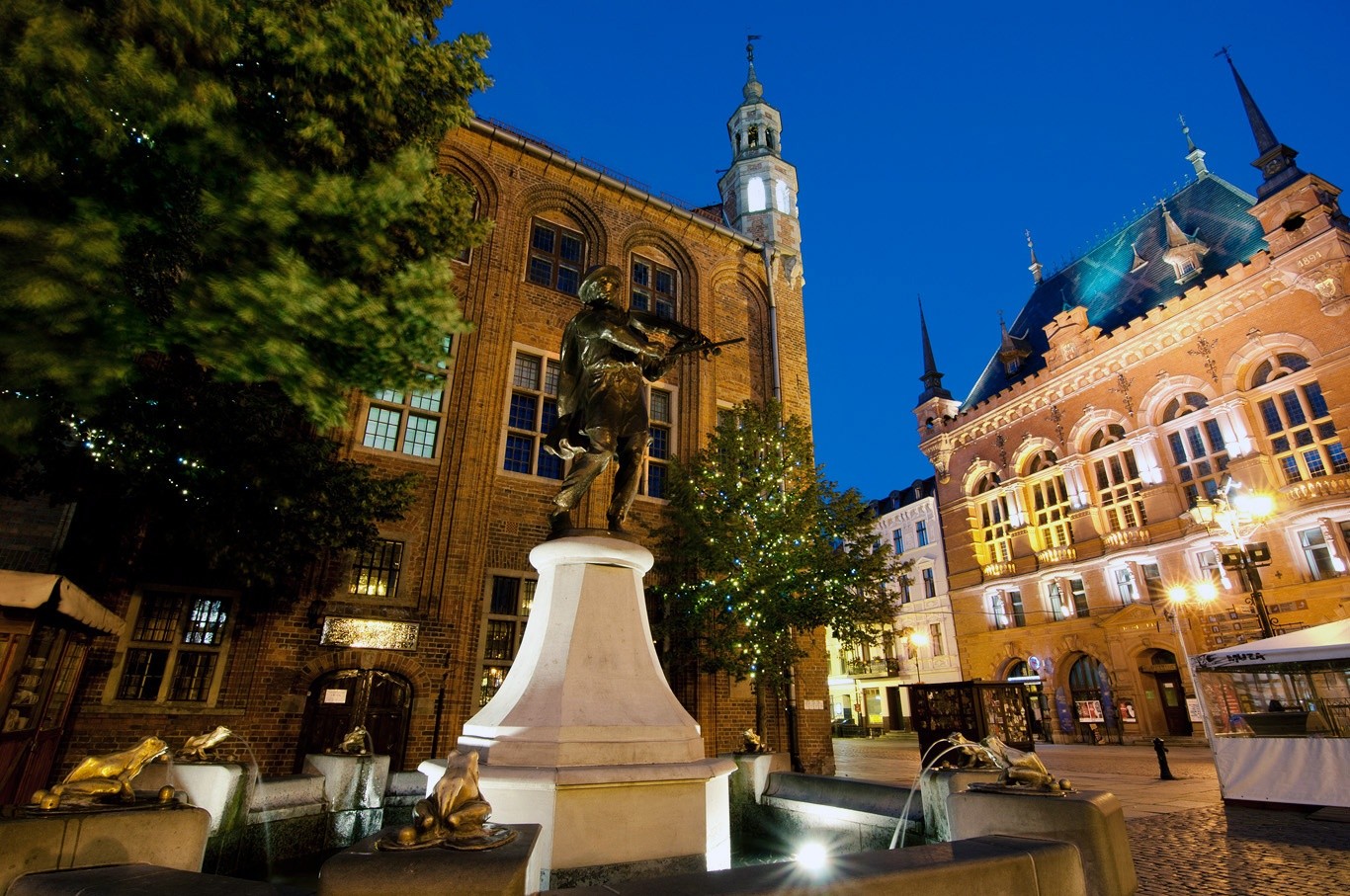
[307,599,326,630]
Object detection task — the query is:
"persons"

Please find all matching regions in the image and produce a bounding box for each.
[1123,702,1135,719]
[545,262,705,535]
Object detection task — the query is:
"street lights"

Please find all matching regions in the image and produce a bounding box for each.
[910,633,930,684]
[1226,493,1276,641]
[1167,578,1219,748]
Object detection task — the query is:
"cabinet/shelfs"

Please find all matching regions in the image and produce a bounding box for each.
[7,667,44,732]
